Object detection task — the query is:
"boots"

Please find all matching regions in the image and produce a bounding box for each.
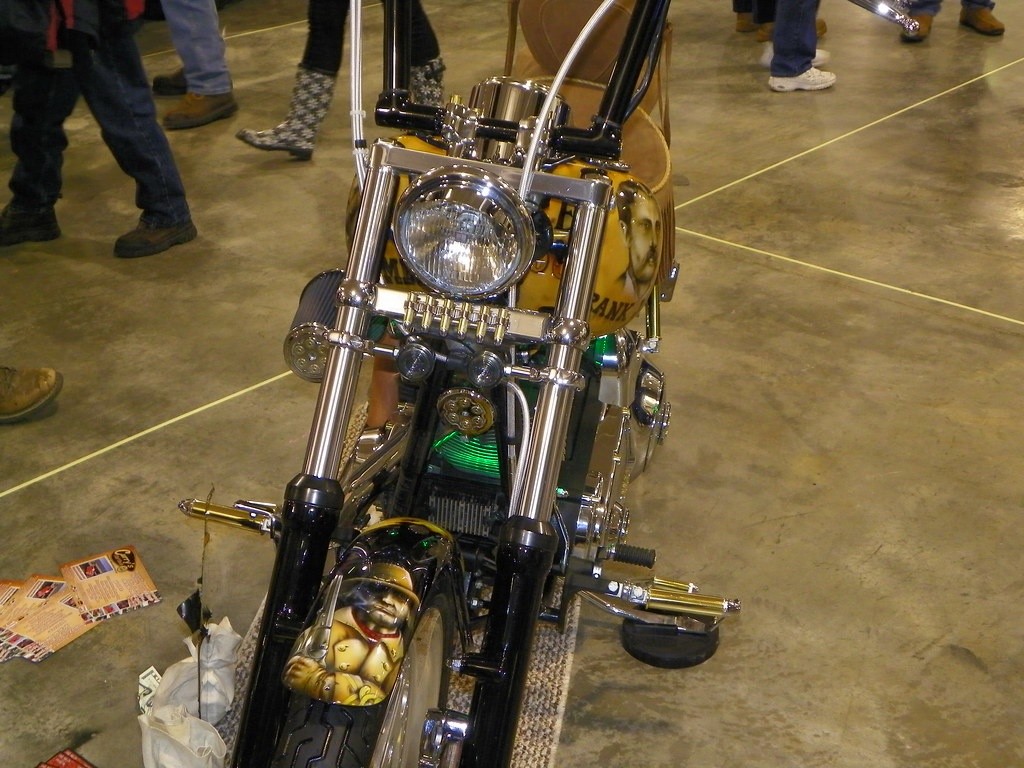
[236,63,338,160]
[401,55,447,142]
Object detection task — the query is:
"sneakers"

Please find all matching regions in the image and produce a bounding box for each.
[900,15,933,39]
[163,92,240,127]
[0,203,61,247]
[759,41,830,68]
[153,67,233,95]
[960,6,1005,35]
[768,67,836,91]
[115,221,197,258]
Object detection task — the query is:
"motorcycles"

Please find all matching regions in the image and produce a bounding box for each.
[175,1,743,767]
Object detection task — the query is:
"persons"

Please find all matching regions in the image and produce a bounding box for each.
[1,0,197,259]
[152,0,241,128]
[733,0,1006,93]
[0,366,64,424]
[236,0,444,160]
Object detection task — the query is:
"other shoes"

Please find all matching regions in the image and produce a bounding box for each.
[756,18,827,42]
[736,12,761,32]
[0,366,63,424]
